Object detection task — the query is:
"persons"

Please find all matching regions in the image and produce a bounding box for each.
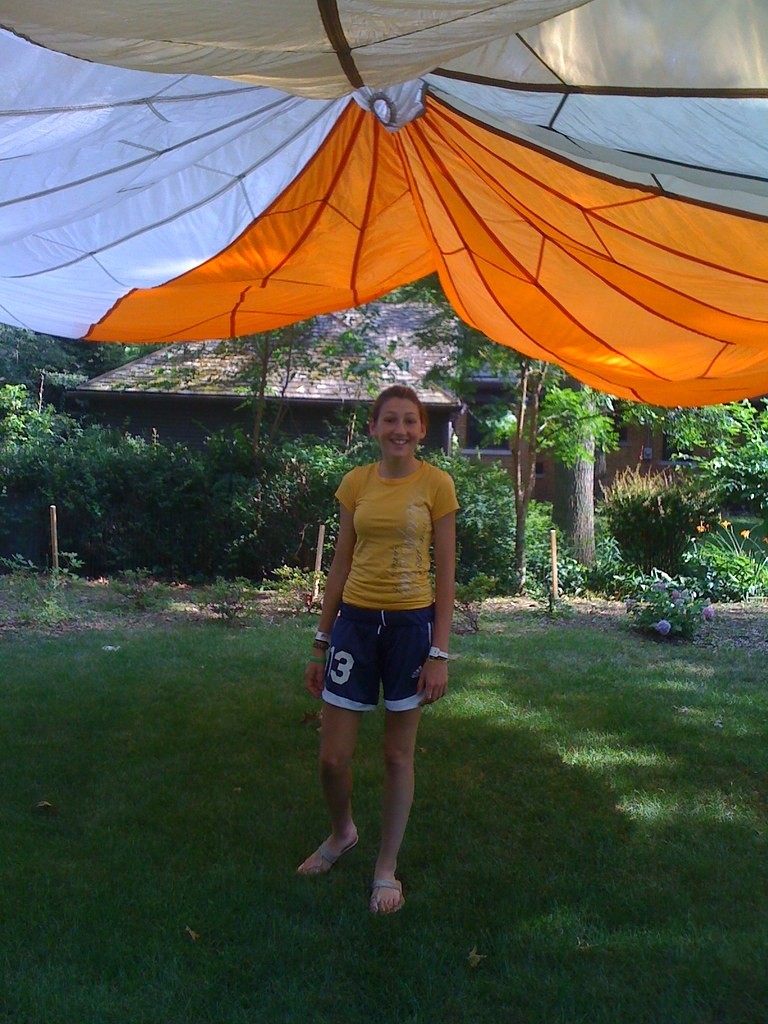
[293,385,459,912]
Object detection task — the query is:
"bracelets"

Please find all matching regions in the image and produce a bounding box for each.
[313,631,331,651]
[310,656,327,662]
[428,646,448,661]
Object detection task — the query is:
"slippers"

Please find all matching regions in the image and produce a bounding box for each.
[369,880,405,914]
[297,835,358,875]
